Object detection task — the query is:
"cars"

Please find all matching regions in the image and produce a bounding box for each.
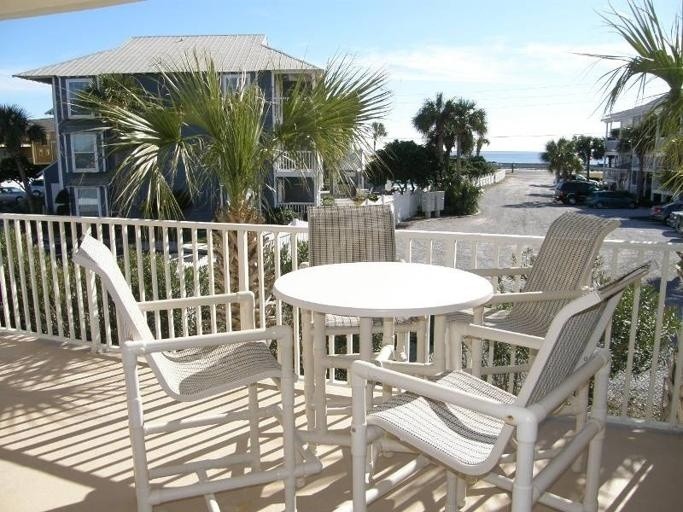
[583,190,637,209]
[0,177,45,205]
[650,201,683,234]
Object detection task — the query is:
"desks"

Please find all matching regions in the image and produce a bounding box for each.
[270,261,494,485]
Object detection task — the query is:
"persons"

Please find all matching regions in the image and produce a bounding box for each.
[510,162,515,173]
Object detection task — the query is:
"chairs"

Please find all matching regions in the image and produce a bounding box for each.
[346,262,652,512]
[73,232,301,512]
[462,211,621,472]
[294,202,412,392]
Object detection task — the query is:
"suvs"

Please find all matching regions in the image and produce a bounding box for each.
[553,181,603,205]
[553,173,598,186]
